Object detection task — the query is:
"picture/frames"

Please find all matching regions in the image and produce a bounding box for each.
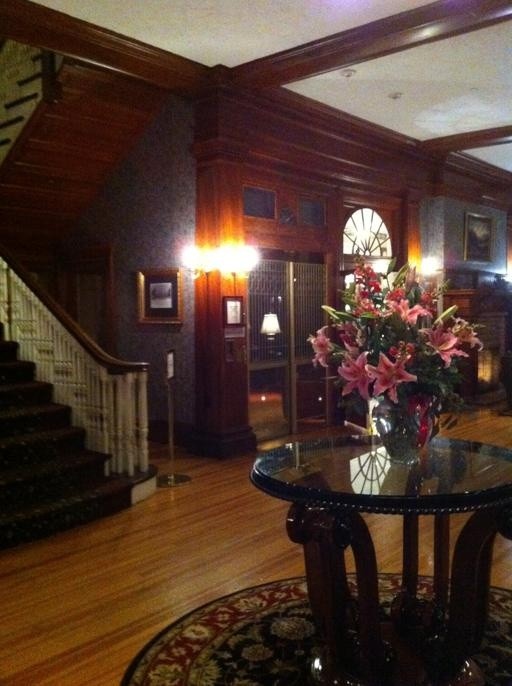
[135,269,183,327]
[464,213,495,263]
[223,294,244,326]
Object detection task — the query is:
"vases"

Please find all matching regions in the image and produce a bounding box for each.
[374,399,433,465]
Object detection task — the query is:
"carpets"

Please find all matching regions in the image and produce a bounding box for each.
[120,574,512,686]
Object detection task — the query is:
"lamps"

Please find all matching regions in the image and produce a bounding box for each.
[259,313,281,341]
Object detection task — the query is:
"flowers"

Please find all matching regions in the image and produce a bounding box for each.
[305,246,486,420]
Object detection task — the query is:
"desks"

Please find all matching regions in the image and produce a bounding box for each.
[249,436,512,686]
